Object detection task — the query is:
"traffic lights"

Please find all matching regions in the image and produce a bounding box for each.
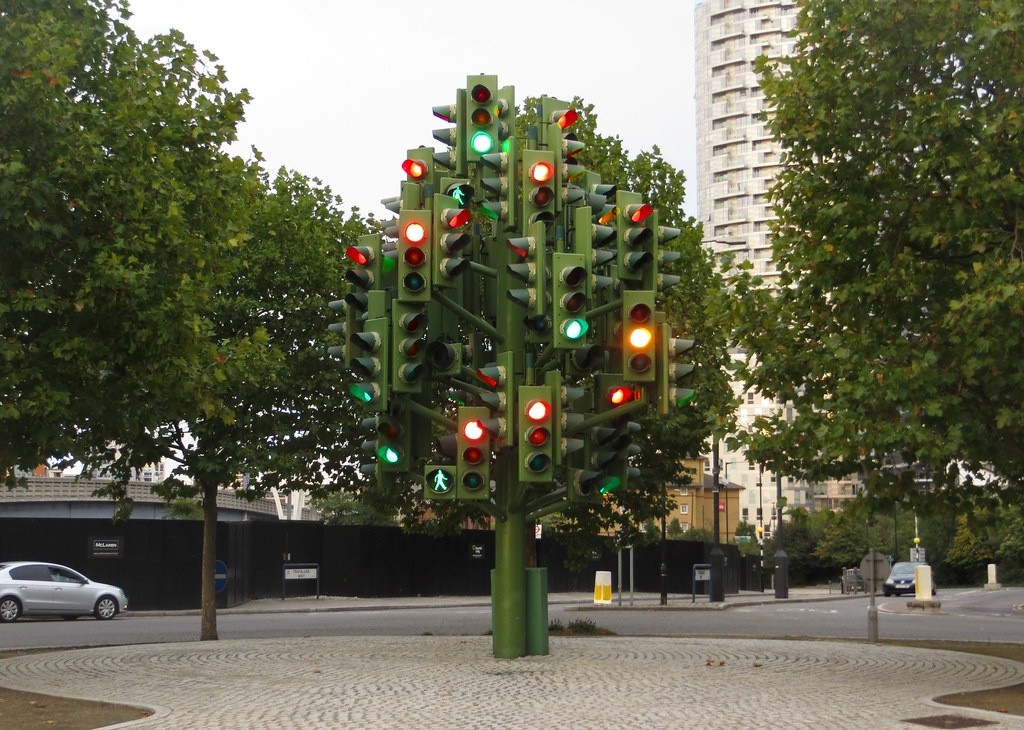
[320,72,698,507]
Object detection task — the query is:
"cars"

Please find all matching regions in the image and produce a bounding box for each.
[883,560,938,598]
[0,559,132,622]
